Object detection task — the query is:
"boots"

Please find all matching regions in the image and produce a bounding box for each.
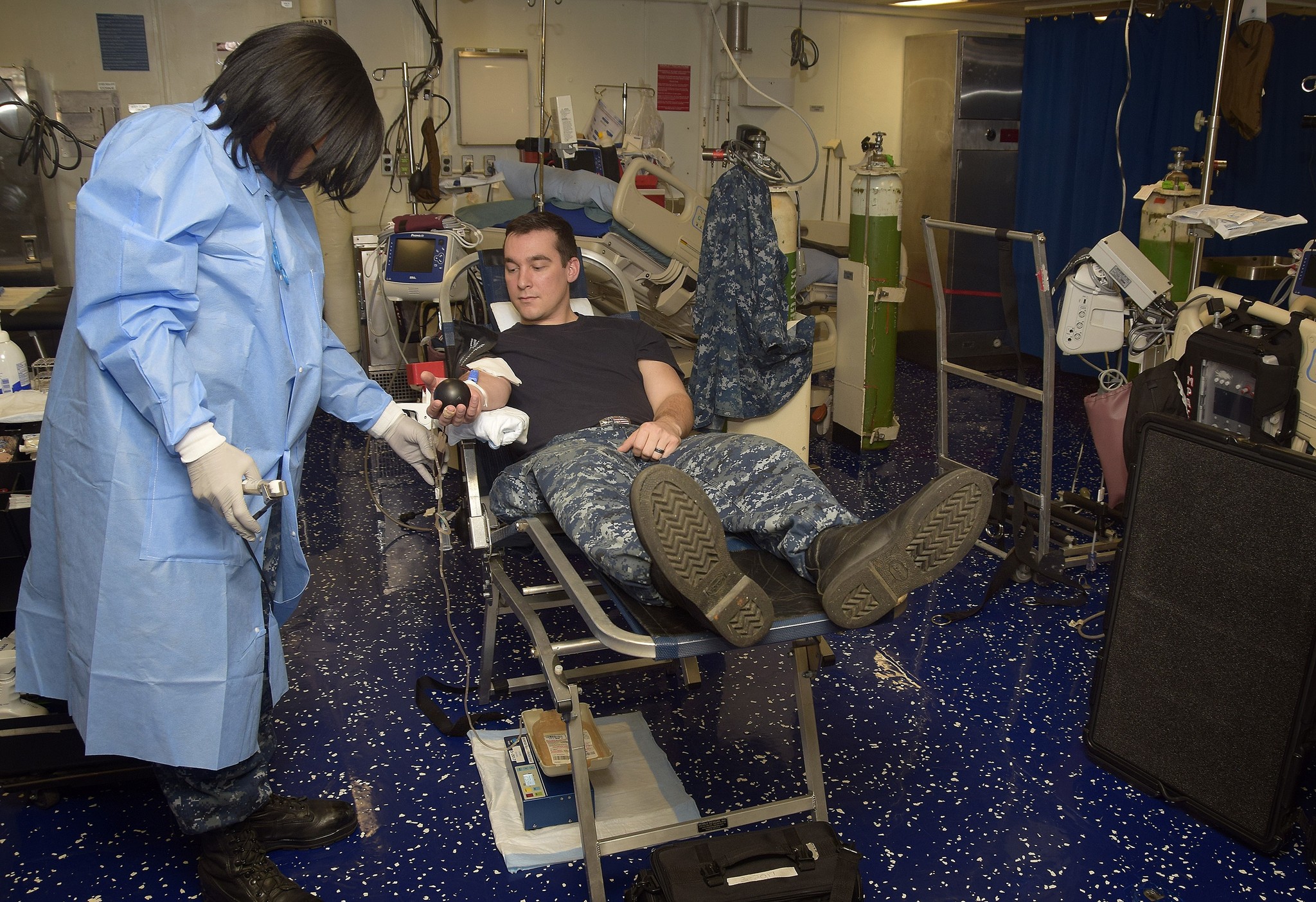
[199,821,325,902]
[629,464,775,647]
[245,792,358,852]
[805,467,993,629]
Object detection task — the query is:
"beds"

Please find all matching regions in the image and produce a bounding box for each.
[454,160,844,392]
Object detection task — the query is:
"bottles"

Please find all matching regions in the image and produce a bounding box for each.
[0,330,31,396]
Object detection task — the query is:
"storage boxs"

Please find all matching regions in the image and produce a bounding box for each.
[625,822,865,902]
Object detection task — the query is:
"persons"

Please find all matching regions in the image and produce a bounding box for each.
[17,19,452,893]
[420,210,991,651]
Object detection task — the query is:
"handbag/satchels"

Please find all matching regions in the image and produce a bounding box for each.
[626,821,864,902]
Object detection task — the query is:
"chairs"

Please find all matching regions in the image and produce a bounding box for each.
[411,245,920,899]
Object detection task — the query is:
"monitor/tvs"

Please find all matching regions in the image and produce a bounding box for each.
[383,230,469,303]
